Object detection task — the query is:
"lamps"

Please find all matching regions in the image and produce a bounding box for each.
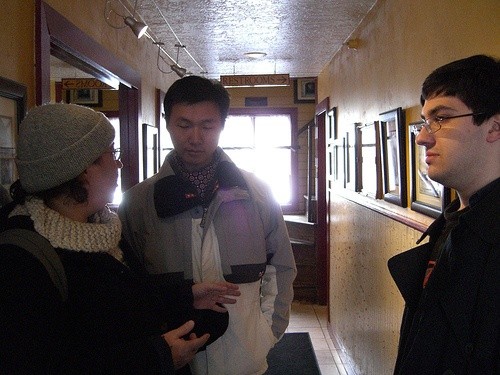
[152,40,187,78]
[104,0,149,40]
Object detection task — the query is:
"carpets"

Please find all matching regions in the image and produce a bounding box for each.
[262,332,321,375]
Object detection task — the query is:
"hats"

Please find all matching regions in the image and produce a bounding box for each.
[14,103,115,192]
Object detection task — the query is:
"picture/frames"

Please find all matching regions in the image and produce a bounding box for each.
[293,77,318,104]
[326,106,382,200]
[142,123,159,180]
[408,121,451,219]
[65,88,103,108]
[0,75,28,214]
[378,106,408,208]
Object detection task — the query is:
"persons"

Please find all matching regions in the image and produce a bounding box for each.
[113,74,297,375]
[387,55,500,375]
[0,101,242,375]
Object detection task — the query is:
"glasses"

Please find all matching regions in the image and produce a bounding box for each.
[414,111,492,134]
[103,148,124,161]
